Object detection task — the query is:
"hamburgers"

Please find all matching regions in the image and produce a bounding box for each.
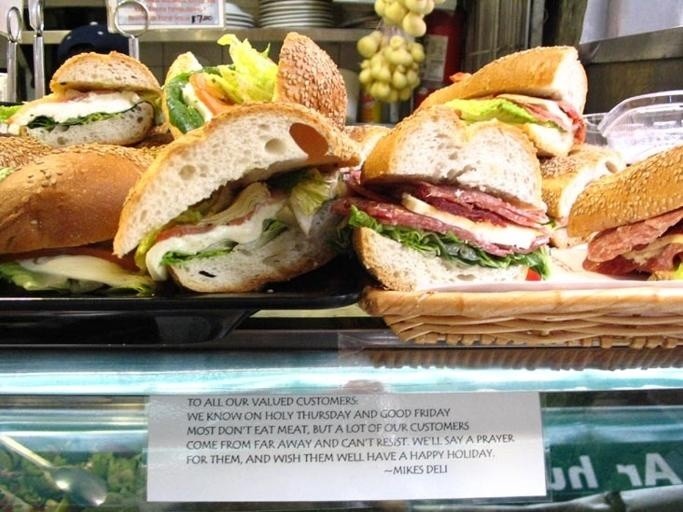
[1,31,683,290]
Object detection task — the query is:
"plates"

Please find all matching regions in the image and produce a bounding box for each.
[218,0,371,32]
